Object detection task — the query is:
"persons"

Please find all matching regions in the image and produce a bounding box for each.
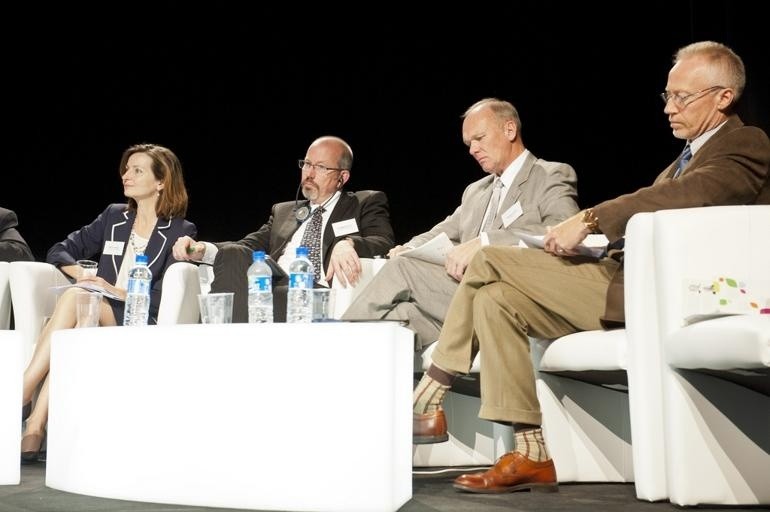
[172,136,394,322]
[0,204,35,263]
[411,41,770,496]
[24,142,197,467]
[337,99,587,355]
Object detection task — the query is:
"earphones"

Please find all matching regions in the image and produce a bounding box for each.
[339,177,343,184]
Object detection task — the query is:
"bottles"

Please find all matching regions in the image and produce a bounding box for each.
[123,256,153,324]
[248,252,272,323]
[286,246,315,324]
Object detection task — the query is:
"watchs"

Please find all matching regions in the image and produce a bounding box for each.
[582,205,600,232]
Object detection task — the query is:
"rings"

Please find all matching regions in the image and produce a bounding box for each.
[555,249,564,253]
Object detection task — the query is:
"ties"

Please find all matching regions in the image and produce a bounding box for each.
[300,206,327,280]
[482,179,504,233]
[671,149,692,178]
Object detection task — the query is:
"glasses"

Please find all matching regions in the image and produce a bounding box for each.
[299,160,350,174]
[661,86,736,107]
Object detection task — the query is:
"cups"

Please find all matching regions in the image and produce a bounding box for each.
[311,288,335,318]
[77,259,99,278]
[74,291,102,328]
[198,292,234,323]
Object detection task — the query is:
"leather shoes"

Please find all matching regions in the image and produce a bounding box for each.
[453,452,559,494]
[413,409,448,444]
[21,429,46,464]
